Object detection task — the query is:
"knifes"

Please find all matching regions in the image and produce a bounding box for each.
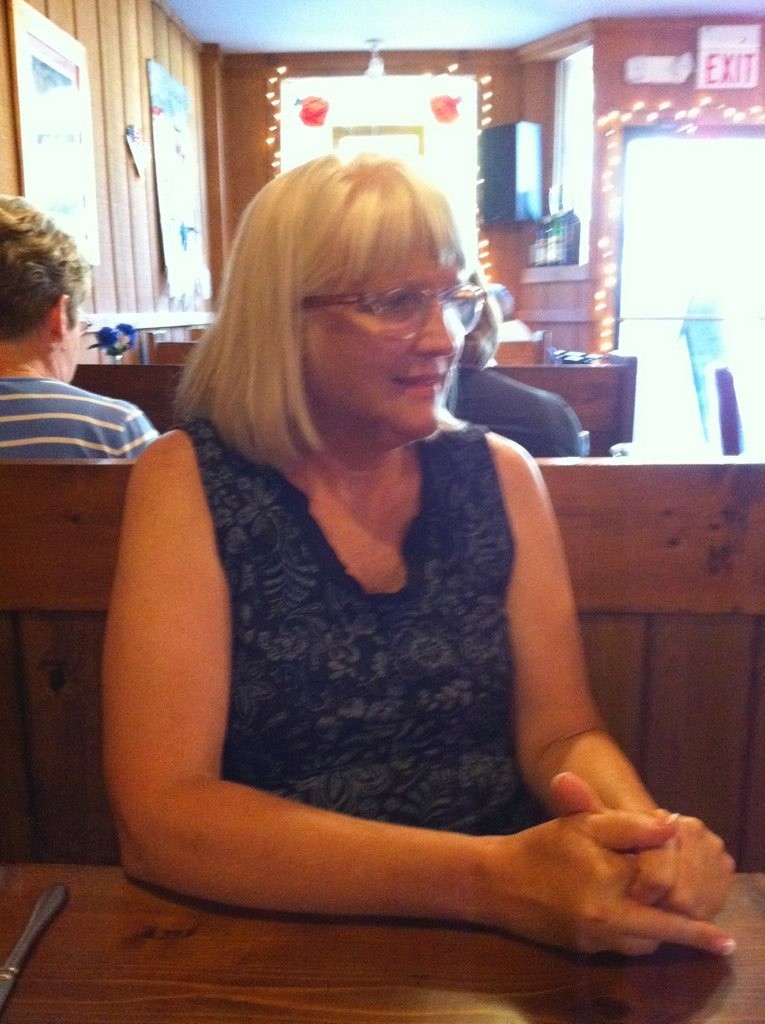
[0,883,67,1008]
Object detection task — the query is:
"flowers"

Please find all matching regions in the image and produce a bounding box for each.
[88,323,135,355]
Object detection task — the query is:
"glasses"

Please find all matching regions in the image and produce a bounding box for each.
[300,286,487,342]
[69,306,93,333]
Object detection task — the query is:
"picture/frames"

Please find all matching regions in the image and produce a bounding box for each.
[6,0,100,264]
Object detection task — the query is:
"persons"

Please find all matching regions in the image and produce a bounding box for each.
[102,154,739,959]
[441,272,587,458]
[0,196,162,461]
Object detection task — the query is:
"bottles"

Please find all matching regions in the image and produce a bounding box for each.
[535,206,580,267]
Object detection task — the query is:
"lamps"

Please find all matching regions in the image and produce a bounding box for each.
[626,52,693,85]
[362,38,384,77]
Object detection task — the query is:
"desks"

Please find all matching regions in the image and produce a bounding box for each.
[0,861,765,1024]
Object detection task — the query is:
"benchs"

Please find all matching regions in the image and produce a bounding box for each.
[71,334,638,460]
[1,459,764,874]
